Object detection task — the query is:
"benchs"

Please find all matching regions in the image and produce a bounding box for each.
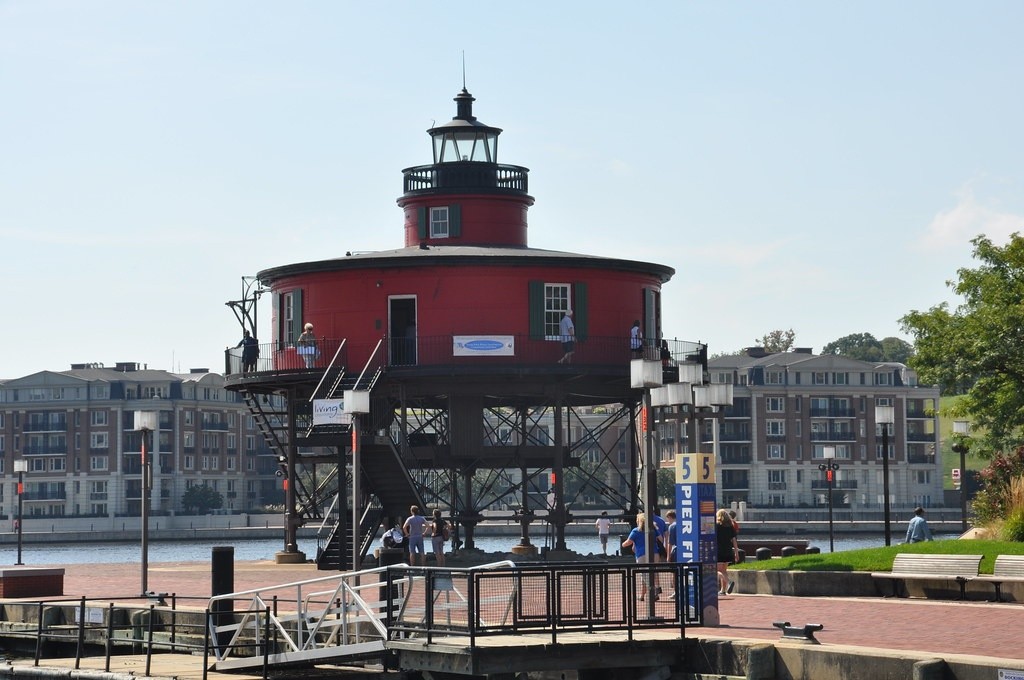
[737,540,810,556]
[973,554,1024,603]
[0,567,65,598]
[871,552,985,601]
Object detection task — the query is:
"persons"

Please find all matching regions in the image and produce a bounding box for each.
[403,505,432,573]
[14,519,18,533]
[596,511,610,555]
[557,309,575,365]
[621,509,740,600]
[905,507,933,544]
[631,320,642,359]
[432,510,451,572]
[231,330,260,379]
[298,322,319,374]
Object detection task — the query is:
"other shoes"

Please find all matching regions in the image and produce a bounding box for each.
[635,595,644,601]
[727,580,735,594]
[667,591,676,600]
[654,593,659,601]
[717,591,726,596]
[420,569,428,574]
[655,587,662,594]
[407,569,414,573]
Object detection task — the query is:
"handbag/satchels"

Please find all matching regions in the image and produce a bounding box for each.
[443,527,450,541]
[653,527,667,559]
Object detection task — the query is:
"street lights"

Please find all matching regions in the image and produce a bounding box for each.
[631,357,663,618]
[954,419,969,532]
[343,389,370,604]
[650,364,735,452]
[823,445,837,552]
[134,412,156,594]
[14,458,28,563]
[875,405,894,546]
